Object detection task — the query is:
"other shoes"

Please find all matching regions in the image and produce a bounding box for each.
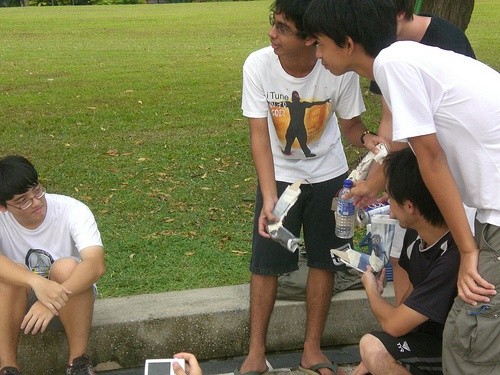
[67,354,94,374]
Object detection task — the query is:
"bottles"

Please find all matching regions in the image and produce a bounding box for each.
[335,180,355,239]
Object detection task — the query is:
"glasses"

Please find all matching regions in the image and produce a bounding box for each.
[5,176,49,211]
[269,13,309,36]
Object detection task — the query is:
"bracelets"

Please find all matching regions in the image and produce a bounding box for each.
[360,130,378,144]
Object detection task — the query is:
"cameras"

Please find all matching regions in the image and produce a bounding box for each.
[144,357,185,375]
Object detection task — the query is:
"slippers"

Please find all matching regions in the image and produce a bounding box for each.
[232,367,269,375]
[297,357,349,374]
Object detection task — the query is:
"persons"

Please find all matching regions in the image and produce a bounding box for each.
[369,0,476,138]
[233,0,391,375]
[352,147,460,375]
[173,352,202,375]
[0,154,104,375]
[306,0,500,375]
